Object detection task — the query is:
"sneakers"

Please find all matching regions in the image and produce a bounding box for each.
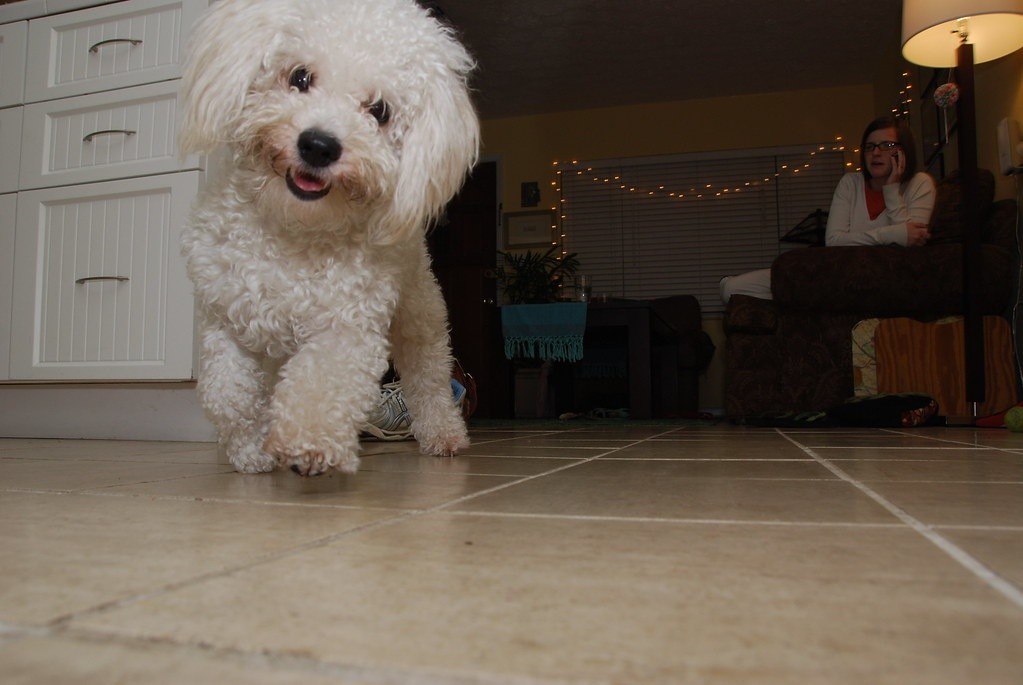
[358,367,467,441]
[752,406,799,426]
[790,407,833,426]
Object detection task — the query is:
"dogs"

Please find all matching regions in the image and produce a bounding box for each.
[174,0,479,479]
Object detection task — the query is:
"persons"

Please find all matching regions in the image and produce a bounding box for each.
[719,117,936,313]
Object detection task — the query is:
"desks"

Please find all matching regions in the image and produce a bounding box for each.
[491,297,700,418]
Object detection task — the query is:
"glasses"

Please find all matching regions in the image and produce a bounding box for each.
[861,141,903,152]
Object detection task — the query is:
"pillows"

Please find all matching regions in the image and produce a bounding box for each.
[827,393,939,429]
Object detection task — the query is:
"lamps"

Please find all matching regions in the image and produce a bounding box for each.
[900,0,1023,69]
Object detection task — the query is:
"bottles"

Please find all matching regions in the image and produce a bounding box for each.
[574,273,591,303]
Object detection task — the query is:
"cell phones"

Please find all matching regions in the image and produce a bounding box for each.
[893,148,903,163]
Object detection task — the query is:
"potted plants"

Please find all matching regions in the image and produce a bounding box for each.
[485,243,582,305]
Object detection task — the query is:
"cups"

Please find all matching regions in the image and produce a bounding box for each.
[596,293,606,303]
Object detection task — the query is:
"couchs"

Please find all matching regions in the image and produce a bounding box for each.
[723,165,1017,429]
